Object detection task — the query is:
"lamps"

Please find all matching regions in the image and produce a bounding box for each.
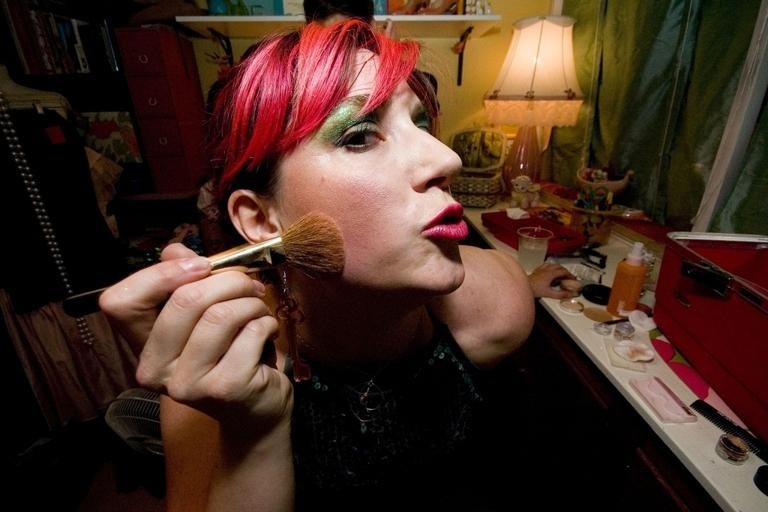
[483,16,586,195]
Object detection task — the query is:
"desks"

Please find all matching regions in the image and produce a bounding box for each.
[460,192,768,511]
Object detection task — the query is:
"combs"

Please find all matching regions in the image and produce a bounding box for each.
[690,400,768,462]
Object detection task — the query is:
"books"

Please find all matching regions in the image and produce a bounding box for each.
[0,0,120,74]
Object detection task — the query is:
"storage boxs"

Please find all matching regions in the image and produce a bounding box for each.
[651,231,768,448]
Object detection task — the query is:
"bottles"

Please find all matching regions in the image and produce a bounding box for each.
[639,250,657,297]
[637,279,659,314]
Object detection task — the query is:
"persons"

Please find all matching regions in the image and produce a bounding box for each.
[531,261,579,308]
[98,18,577,512]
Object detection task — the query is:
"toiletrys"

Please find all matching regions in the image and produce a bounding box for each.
[715,433,750,466]
[560,279,583,297]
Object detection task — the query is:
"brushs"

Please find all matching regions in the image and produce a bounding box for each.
[62,210,346,318]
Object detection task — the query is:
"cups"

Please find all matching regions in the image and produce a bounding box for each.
[517,228,552,275]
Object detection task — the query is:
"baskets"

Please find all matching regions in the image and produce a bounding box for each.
[452,172,501,207]
